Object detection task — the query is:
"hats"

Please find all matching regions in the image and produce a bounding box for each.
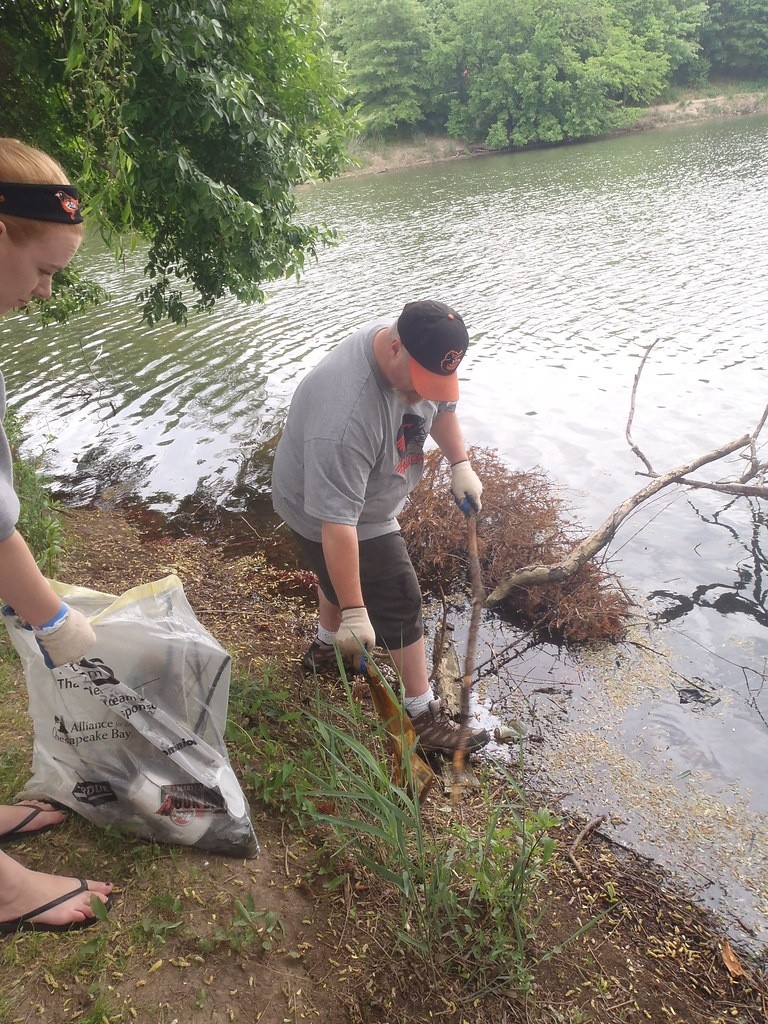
[397,299,469,401]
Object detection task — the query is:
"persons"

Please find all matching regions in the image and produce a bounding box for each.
[270,300,493,756]
[0,136,119,933]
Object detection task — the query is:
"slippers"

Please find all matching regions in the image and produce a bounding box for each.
[0,805,61,844]
[0,876,116,933]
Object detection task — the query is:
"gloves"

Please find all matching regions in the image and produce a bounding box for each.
[450,460,483,516]
[335,606,376,674]
[32,602,96,669]
[0,603,33,631]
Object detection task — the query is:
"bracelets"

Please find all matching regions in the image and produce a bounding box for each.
[37,602,67,630]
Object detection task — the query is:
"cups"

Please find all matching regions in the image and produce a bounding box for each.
[127,771,213,846]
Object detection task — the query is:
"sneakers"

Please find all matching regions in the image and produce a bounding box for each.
[405,694,490,755]
[302,636,380,676]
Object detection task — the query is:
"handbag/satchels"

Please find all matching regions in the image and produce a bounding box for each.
[0,574,261,861]
